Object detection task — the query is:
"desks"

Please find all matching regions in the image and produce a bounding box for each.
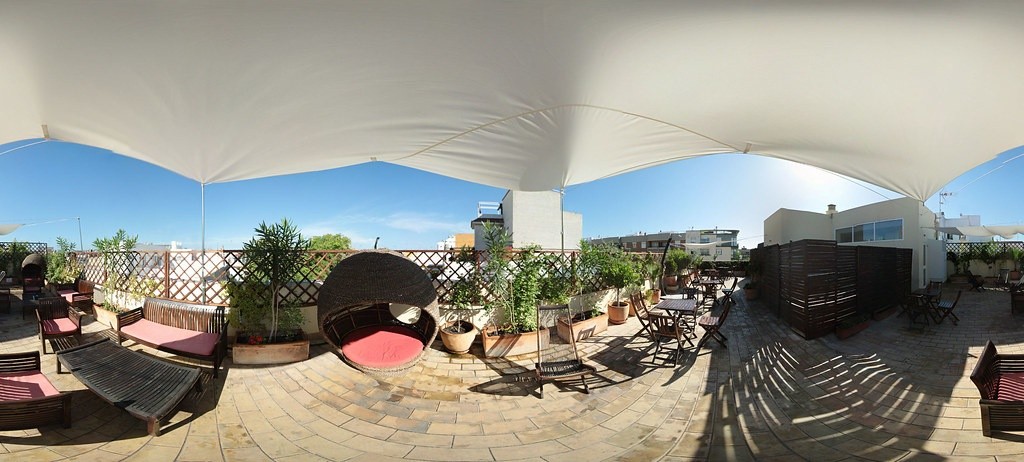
[56,337,204,438]
[912,288,942,324]
[704,269,716,281]
[699,279,723,307]
[656,298,698,347]
[21,293,52,320]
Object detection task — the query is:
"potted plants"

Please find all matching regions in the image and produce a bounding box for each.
[92,272,134,331]
[539,254,610,343]
[743,264,762,300]
[459,219,545,359]
[947,251,970,284]
[1007,247,1024,280]
[835,313,871,338]
[576,236,639,325]
[624,251,652,317]
[641,250,661,304]
[439,282,478,356]
[663,248,702,292]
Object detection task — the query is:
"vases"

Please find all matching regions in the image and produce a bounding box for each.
[232,327,310,365]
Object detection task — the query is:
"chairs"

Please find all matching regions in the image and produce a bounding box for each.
[893,269,1024,335]
[23,284,41,294]
[534,303,596,399]
[0,288,11,315]
[629,267,738,368]
[34,296,87,355]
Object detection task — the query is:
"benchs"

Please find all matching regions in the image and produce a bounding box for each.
[0,350,72,431]
[56,277,95,312]
[970,340,1024,437]
[115,298,230,379]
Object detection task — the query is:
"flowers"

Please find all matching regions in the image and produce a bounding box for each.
[219,216,312,345]
